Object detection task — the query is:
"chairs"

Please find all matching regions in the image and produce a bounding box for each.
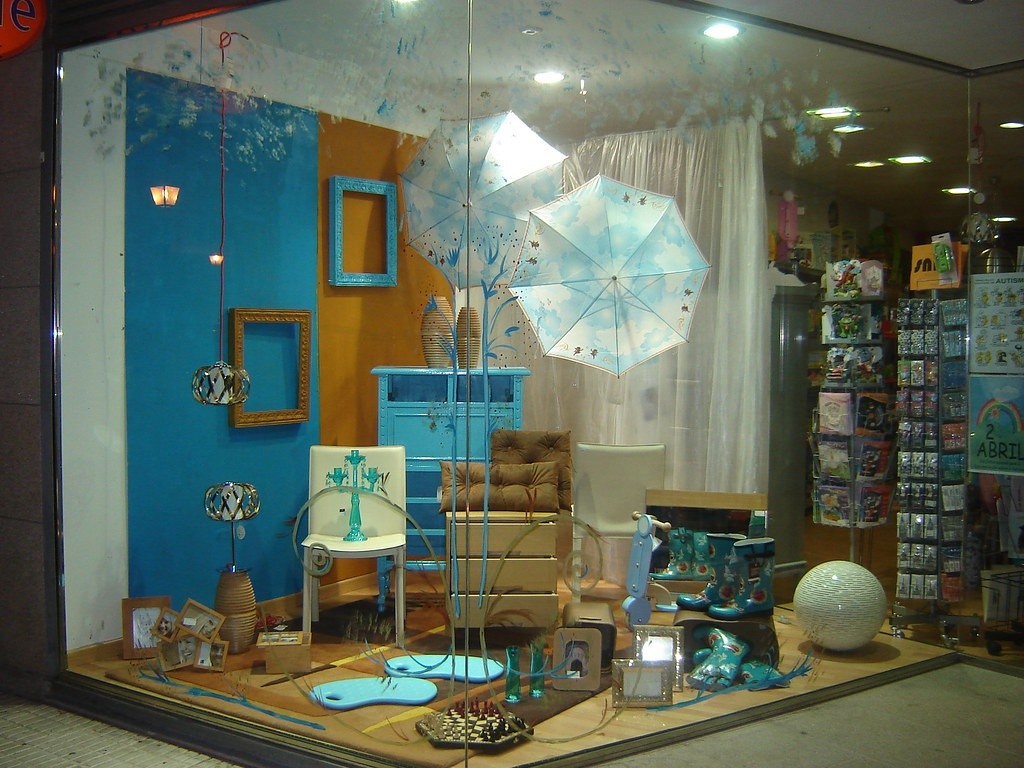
[299,444,410,647]
[569,441,667,605]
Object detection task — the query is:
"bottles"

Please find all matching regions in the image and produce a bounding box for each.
[505,645,522,704]
[530,642,544,699]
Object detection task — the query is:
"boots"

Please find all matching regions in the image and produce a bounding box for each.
[649,529,711,581]
[676,534,747,611]
[686,624,791,693]
[707,538,776,620]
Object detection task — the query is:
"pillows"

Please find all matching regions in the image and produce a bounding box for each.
[491,428,573,510]
[439,459,561,514]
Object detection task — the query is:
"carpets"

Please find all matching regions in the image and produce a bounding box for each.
[103,592,631,767]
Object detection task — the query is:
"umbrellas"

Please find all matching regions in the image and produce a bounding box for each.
[508,171,713,378]
[398,109,570,292]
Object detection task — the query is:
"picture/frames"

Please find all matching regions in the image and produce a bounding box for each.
[552,623,686,708]
[121,595,304,674]
[328,174,398,287]
[228,306,311,427]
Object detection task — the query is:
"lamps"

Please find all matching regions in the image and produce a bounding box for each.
[189,28,256,409]
[203,479,262,655]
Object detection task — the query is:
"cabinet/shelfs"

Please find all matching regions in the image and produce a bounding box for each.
[369,365,530,614]
[444,510,557,645]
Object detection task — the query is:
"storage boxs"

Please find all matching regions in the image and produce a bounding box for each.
[267,632,312,672]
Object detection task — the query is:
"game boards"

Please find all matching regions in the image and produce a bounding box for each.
[414,707,534,750]
